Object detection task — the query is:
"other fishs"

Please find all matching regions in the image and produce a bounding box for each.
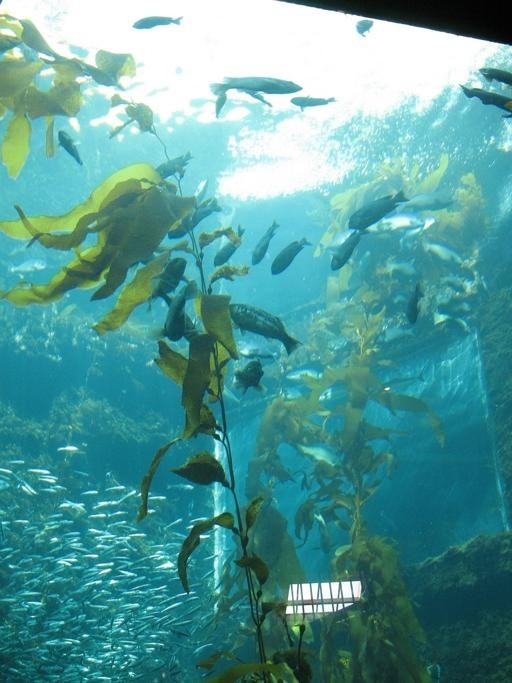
[2,14,510,677]
